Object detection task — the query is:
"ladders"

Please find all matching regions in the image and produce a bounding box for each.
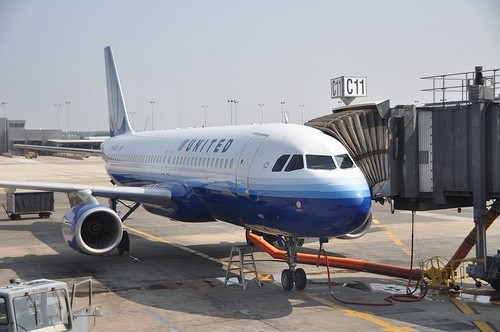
[224,246,262,289]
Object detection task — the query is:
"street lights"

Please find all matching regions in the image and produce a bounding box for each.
[65,101,71,140]
[259,103,264,124]
[54,103,61,139]
[299,104,305,124]
[150,101,155,130]
[228,99,233,126]
[234,100,239,125]
[280,101,286,123]
[202,104,208,127]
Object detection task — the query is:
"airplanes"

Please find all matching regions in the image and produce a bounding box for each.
[0,44,375,291]
